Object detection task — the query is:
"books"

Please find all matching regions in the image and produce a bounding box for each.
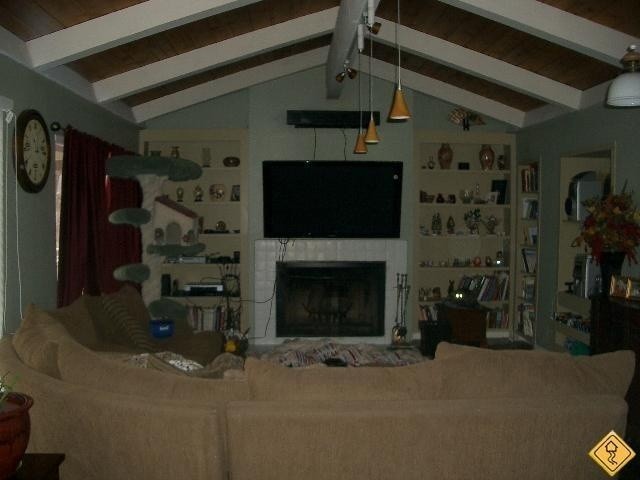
[518,165,540,339]
[419,273,510,330]
[187,305,226,332]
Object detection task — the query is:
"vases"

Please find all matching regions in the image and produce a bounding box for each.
[0,371,34,480]
[479,144,495,171]
[599,253,626,285]
[437,141,454,170]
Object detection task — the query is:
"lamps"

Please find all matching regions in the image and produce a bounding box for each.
[606,43,640,107]
[335,0,411,154]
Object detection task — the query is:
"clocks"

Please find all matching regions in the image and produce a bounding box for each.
[12,109,52,194]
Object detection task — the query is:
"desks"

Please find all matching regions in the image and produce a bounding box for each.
[586,293,639,480]
[13,453,65,480]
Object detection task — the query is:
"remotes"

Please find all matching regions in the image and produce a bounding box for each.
[326,357,347,367]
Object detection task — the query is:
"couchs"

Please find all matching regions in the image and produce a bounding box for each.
[1,285,640,480]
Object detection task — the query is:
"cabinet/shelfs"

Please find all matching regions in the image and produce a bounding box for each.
[407,130,517,341]
[139,128,249,338]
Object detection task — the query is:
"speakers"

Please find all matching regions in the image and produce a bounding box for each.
[572,254,602,299]
[565,171,600,222]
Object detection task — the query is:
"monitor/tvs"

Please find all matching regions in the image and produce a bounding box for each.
[263,161,403,239]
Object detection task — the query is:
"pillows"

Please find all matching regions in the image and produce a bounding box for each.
[51,299,98,347]
[102,295,157,351]
[434,341,635,398]
[55,336,245,396]
[14,304,69,375]
[244,354,456,399]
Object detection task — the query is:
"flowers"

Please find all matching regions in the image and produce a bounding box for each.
[571,180,640,267]
